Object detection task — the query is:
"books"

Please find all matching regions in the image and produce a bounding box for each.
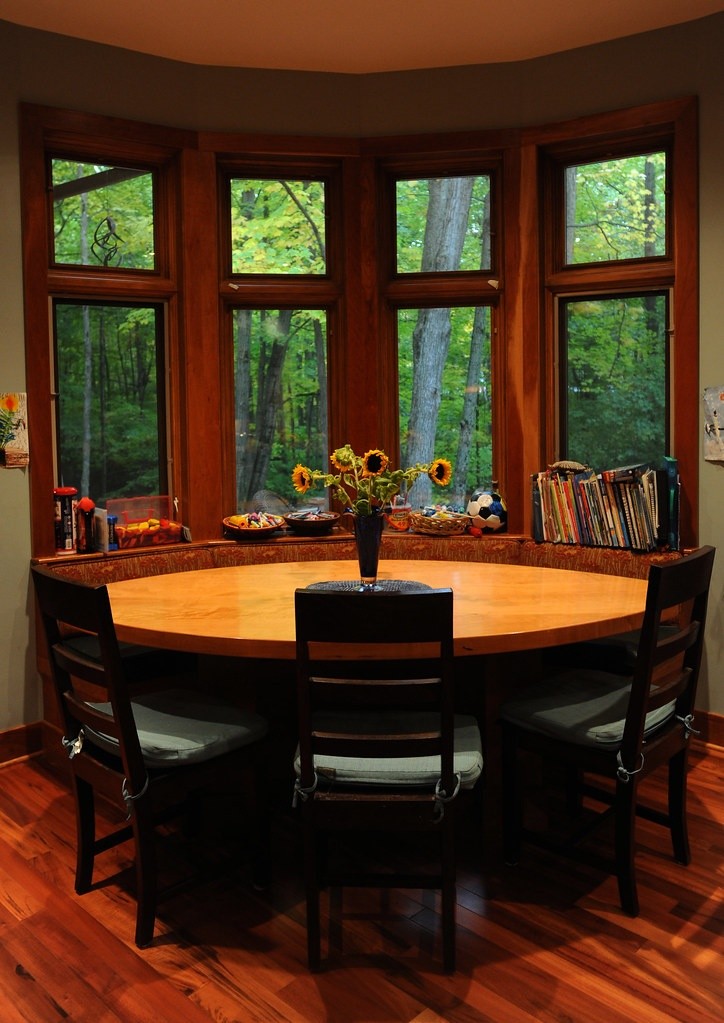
[530,457,678,551]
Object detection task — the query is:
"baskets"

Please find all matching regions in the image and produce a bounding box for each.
[409,505,470,535]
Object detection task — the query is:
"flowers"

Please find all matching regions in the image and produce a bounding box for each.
[291,443,454,518]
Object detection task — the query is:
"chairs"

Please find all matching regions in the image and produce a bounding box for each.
[295,579,483,969]
[28,562,280,948]
[496,544,716,920]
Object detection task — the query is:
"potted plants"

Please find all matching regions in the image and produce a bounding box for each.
[343,506,385,577]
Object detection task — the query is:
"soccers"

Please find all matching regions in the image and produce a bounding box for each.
[465,487,506,530]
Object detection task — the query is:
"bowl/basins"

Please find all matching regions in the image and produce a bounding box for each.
[222,513,284,537]
[283,512,339,529]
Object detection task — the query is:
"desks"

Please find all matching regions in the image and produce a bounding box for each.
[96,558,681,857]
